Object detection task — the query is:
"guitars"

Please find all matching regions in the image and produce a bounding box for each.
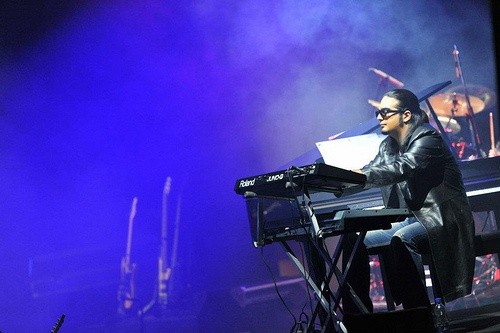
[115,176,175,316]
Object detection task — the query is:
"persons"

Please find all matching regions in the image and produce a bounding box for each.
[334,88,475,333]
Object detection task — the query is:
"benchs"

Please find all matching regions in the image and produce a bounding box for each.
[367,230,500,310]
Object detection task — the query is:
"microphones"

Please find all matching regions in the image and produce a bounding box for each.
[368,68,404,89]
[453,47,461,78]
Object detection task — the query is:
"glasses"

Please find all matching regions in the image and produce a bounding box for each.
[375,108,400,117]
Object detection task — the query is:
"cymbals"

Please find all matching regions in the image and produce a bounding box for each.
[425,86,497,134]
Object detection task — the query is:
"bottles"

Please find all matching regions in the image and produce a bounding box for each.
[435,298,448,333]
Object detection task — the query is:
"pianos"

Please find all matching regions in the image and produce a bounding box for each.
[232,162,415,333]
